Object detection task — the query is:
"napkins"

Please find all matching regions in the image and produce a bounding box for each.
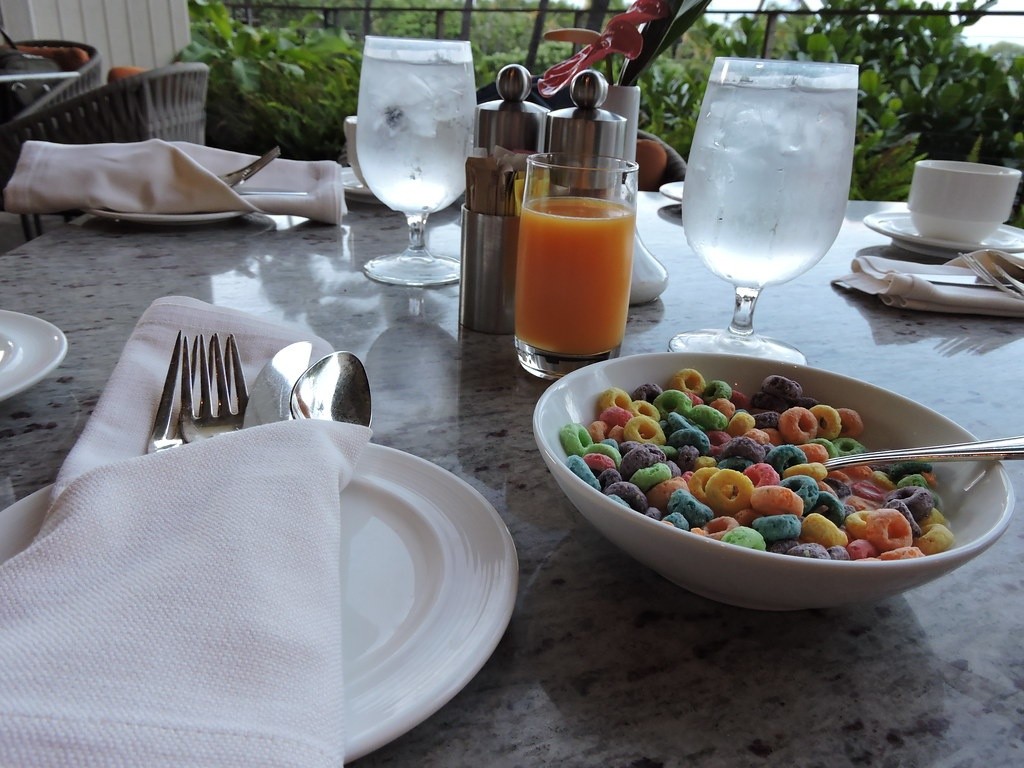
[1,295,373,768]
[5,139,350,226]
[830,249,1024,320]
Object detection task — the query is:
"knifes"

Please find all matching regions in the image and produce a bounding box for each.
[239,191,308,195]
[903,273,1014,287]
[242,340,312,429]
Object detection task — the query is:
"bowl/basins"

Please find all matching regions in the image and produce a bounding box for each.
[533,352,1015,612]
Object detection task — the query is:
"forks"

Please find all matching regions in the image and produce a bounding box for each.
[216,146,281,190]
[146,330,248,470]
[958,252,1024,300]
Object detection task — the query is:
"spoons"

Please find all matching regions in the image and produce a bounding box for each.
[291,351,373,434]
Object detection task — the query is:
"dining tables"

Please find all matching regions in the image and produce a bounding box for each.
[0,199,1024,768]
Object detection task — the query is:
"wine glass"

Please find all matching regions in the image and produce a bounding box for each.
[355,35,477,287]
[668,56,859,367]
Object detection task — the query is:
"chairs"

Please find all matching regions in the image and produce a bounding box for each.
[0,63,209,241]
[0,40,104,120]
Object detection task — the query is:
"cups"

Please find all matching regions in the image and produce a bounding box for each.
[910,160,1022,245]
[513,153,639,380]
[459,204,515,334]
[342,116,370,188]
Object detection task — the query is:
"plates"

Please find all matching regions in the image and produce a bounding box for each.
[660,179,684,202]
[86,208,241,226]
[338,444,519,766]
[340,166,386,204]
[863,212,1023,258]
[0,308,68,401]
